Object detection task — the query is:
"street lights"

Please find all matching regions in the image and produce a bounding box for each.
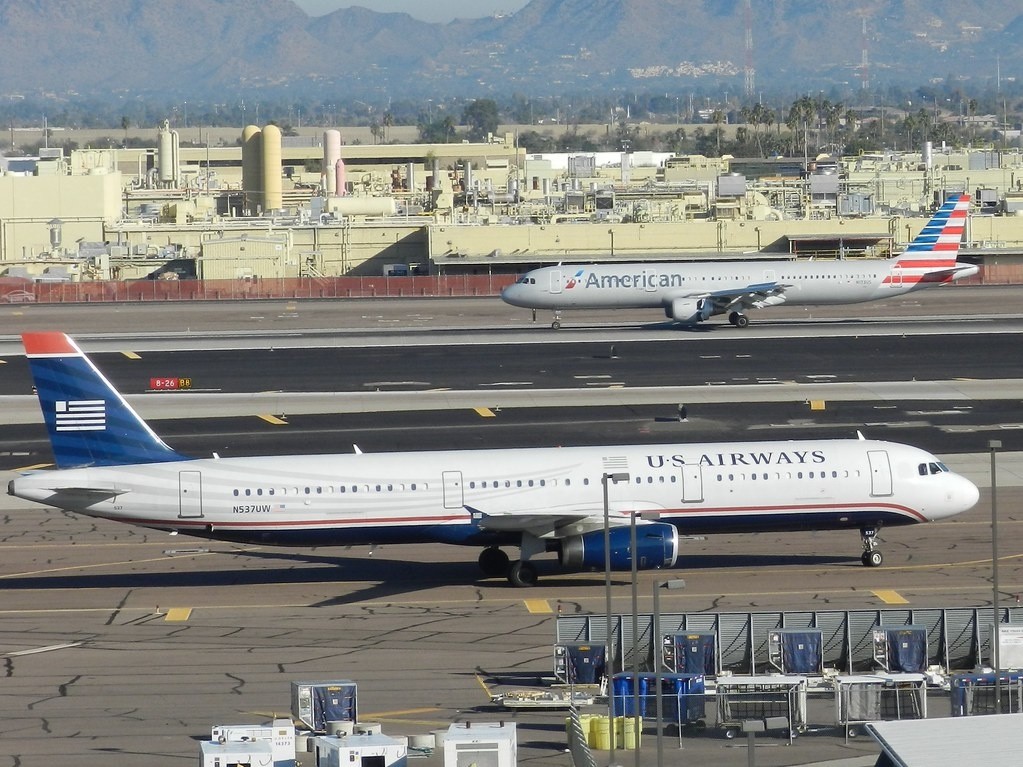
[601,472,630,765]
[654,580,687,767]
[630,510,662,767]
[989,438,1002,715]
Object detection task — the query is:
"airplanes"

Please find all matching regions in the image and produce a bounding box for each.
[501,190,979,330]
[6,330,983,590]
